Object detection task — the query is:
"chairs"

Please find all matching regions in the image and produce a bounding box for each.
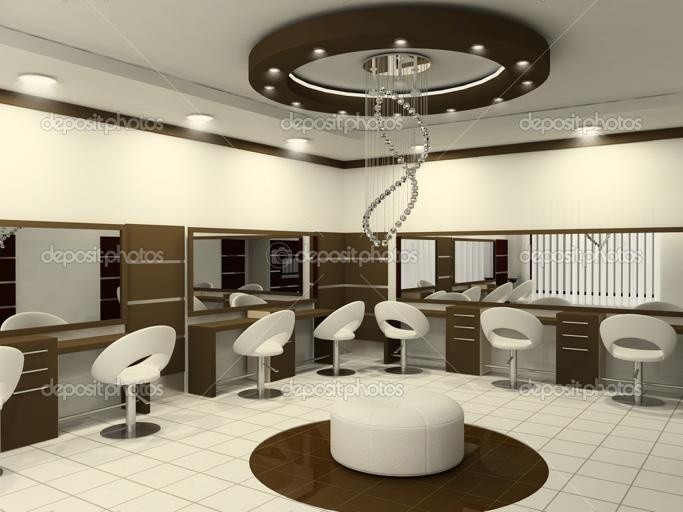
[193,280,268,314]
[0,301,681,457]
[415,276,680,311]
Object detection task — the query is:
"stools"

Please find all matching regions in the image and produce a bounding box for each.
[327,381,466,478]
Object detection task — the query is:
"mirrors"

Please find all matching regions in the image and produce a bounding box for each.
[396,225,681,319]
[187,225,315,319]
[0,220,127,340]
